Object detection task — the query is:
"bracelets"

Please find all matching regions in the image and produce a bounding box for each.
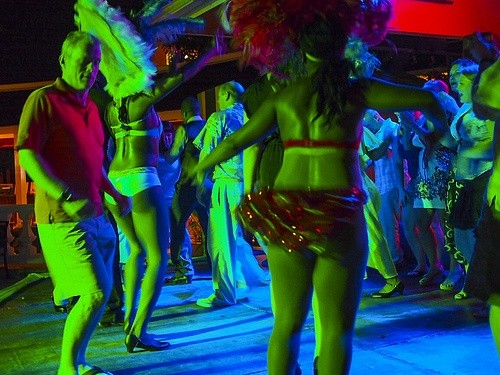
[71,0,222,354]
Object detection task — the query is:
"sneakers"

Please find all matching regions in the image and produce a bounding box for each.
[196,292,236,308]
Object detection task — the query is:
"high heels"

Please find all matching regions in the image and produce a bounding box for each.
[440,270,466,290]
[419,270,443,287]
[123,320,170,353]
[372,280,405,298]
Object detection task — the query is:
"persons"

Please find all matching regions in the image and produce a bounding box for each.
[50,0,248,329]
[15,30,134,375]
[174,0,447,375]
[358,32,500,355]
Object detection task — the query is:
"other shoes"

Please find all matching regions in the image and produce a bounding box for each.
[81,365,113,375]
[454,288,469,299]
[163,272,193,284]
[407,266,427,276]
[50,293,78,312]
[376,262,402,272]
[96,309,124,327]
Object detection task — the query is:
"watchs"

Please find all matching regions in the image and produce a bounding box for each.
[57,185,74,206]
